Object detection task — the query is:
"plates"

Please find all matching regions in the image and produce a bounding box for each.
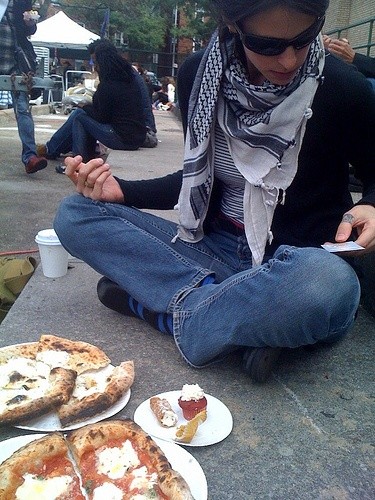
[0,341,132,434]
[133,388,234,448]
[0,431,210,500]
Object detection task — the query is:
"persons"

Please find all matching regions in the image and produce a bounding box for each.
[49,56,63,81]
[55,41,147,175]
[130,62,152,101]
[35,39,158,160]
[321,35,375,90]
[63,61,72,82]
[53,0,375,384]
[0,0,48,174]
[152,76,176,110]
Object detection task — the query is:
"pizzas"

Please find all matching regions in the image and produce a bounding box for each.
[0,432,84,500]
[66,420,196,499]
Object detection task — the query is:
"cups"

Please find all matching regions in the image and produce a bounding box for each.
[33,227,69,279]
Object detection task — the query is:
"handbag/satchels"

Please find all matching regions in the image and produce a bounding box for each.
[13,42,37,76]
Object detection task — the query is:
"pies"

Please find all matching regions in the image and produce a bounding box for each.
[57,357,135,428]
[0,343,39,364]
[35,335,112,375]
[0,351,77,426]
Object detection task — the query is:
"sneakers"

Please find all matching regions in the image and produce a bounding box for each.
[37,144,53,160]
[97,276,174,335]
[222,347,279,384]
[56,165,79,175]
[24,155,48,174]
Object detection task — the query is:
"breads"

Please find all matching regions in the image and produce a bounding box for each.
[174,409,206,442]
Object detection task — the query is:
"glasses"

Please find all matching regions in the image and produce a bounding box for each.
[233,15,326,57]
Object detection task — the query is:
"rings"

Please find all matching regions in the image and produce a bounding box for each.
[342,213,356,227]
[85,181,94,188]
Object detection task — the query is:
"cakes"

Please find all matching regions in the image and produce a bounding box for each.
[150,397,177,427]
[178,381,207,421]
